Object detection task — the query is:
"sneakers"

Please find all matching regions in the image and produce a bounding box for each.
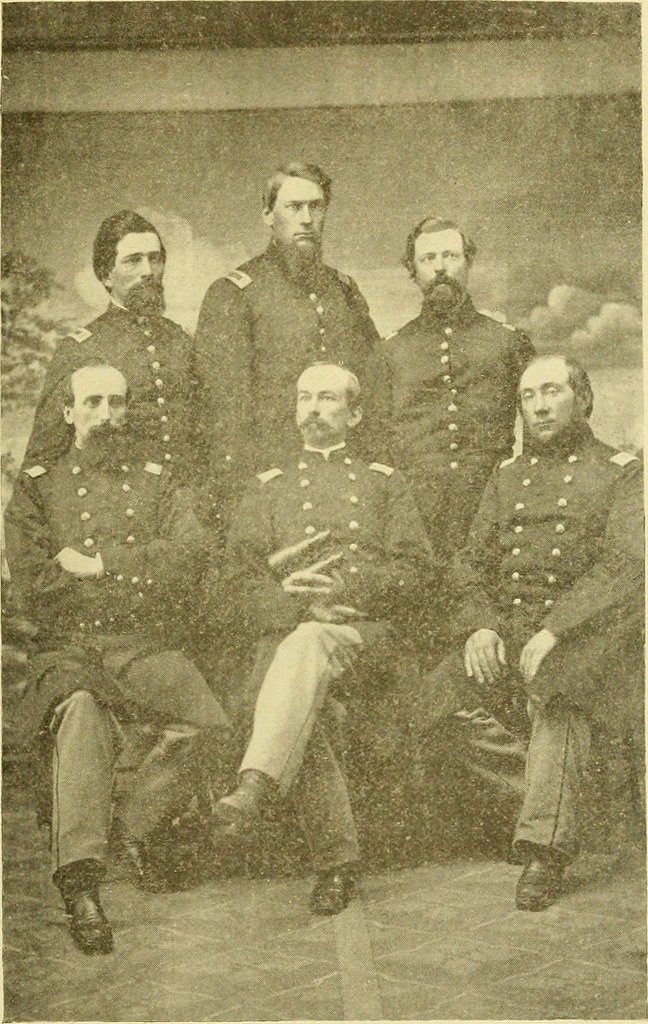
[517,855,564,913]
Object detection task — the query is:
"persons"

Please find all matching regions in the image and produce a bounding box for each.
[211,359,435,915]
[194,157,383,467]
[2,360,233,956]
[15,208,202,485]
[373,215,538,558]
[415,355,646,914]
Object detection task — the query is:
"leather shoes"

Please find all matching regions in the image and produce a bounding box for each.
[64,889,114,954]
[209,769,279,831]
[309,870,349,915]
[109,817,171,894]
